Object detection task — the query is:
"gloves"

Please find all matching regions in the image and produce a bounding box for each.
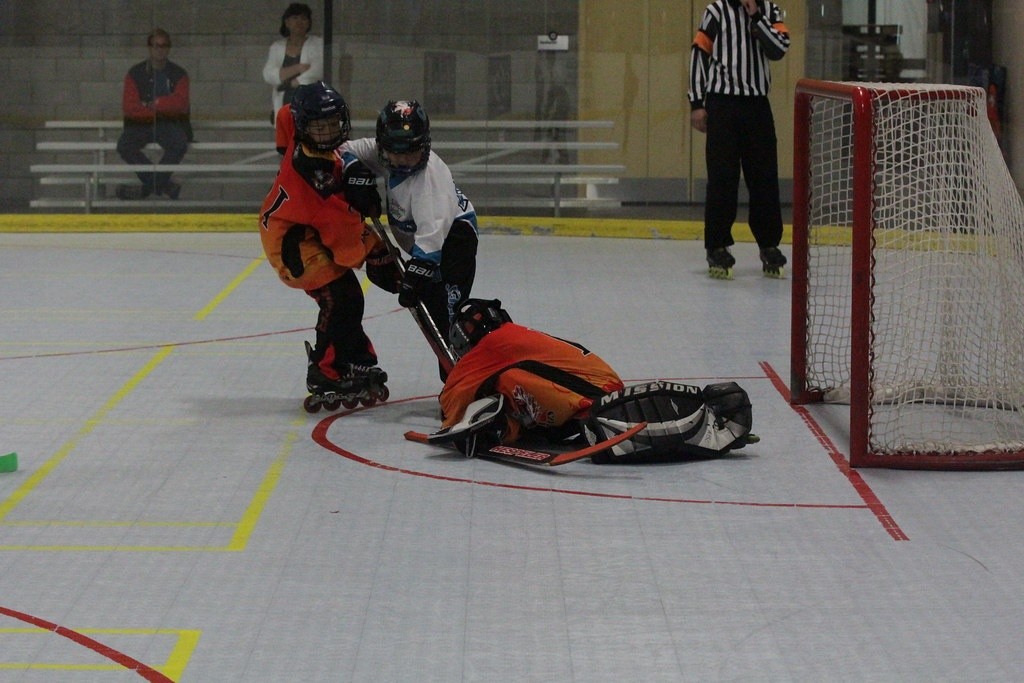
[365,242,401,294]
[399,259,442,307]
[340,160,383,218]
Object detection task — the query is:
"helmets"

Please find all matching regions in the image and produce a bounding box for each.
[376,100,430,173]
[291,79,351,153]
[448,298,513,362]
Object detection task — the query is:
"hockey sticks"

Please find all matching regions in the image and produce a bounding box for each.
[370,217,458,374]
[404,420,649,466]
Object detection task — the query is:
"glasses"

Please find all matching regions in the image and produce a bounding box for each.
[149,43,170,48]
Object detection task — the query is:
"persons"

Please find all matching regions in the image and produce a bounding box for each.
[429,296,760,465]
[687,0,792,280]
[263,3,324,130]
[116,28,193,200]
[259,82,406,414]
[340,99,478,381]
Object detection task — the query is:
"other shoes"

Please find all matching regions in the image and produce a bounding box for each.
[117,183,146,200]
[168,183,181,198]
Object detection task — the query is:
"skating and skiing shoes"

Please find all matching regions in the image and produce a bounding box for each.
[304,363,389,413]
[759,247,787,279]
[706,246,736,279]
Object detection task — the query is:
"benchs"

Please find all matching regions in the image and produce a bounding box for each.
[28,121,628,217]
